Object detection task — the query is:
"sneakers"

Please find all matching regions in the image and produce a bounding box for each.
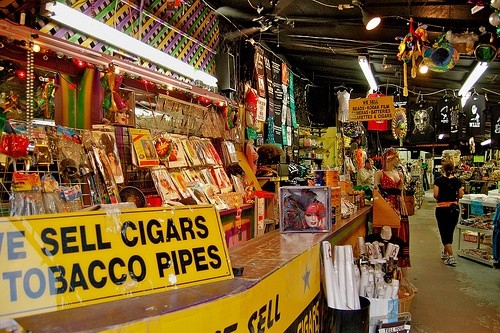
[444,256,457,264]
[441,251,448,260]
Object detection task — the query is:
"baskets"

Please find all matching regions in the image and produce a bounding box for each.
[399,283,415,314]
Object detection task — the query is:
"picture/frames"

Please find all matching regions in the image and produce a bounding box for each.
[278,185,331,233]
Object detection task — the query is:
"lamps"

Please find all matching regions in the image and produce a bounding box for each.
[356,55,379,92]
[454,59,490,98]
[39,0,219,89]
[352,0,381,31]
[418,58,429,74]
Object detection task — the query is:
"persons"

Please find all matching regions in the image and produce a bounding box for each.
[357,158,375,185]
[395,121,405,138]
[105,147,122,177]
[434,161,464,265]
[466,155,473,167]
[169,142,178,161]
[160,175,176,194]
[374,148,419,293]
[116,100,129,126]
